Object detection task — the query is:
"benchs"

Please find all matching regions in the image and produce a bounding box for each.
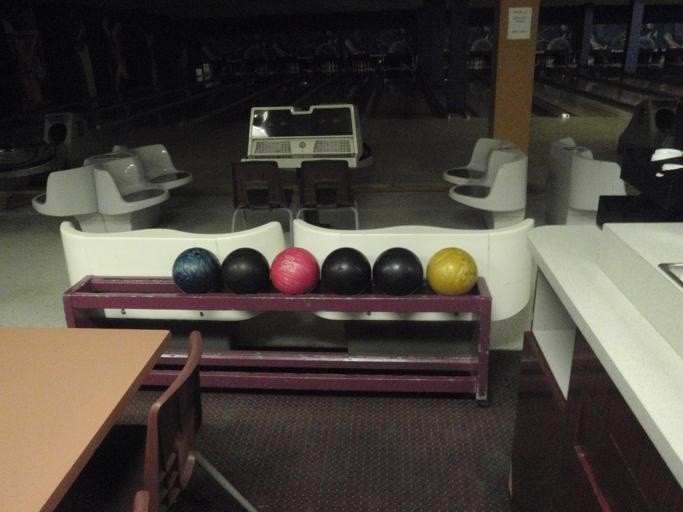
[291,218,536,352]
[59,220,287,350]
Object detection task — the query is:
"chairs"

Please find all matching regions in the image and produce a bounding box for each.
[441,137,527,228]
[55,331,260,512]
[294,160,361,230]
[229,160,296,248]
[32,143,194,235]
[546,136,627,226]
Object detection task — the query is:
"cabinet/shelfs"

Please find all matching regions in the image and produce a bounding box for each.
[508,259,683,512]
[62,274,493,409]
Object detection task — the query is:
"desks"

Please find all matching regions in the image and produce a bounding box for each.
[0,326,172,512]
